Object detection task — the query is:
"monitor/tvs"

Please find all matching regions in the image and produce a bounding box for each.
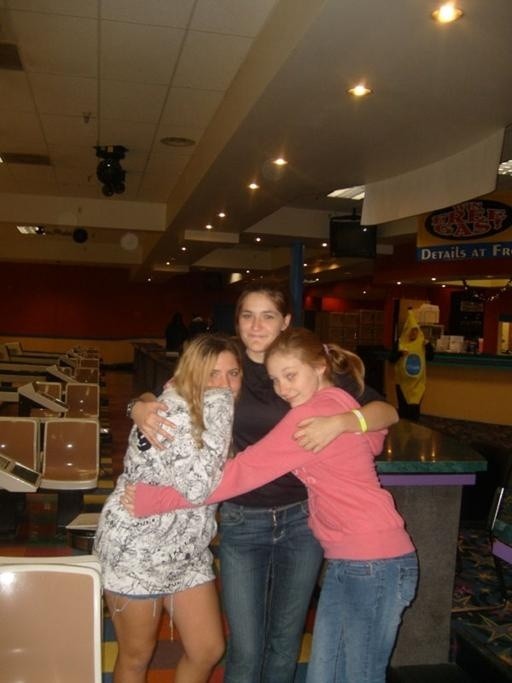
[329,214,377,259]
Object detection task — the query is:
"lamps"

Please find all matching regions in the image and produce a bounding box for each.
[95,145,130,196]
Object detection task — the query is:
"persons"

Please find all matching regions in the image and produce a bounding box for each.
[127,282,399,683]
[93,336,243,683]
[165,311,216,349]
[390,324,436,422]
[121,328,420,683]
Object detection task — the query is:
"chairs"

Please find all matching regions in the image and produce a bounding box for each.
[0,345,110,541]
[0,555,106,683]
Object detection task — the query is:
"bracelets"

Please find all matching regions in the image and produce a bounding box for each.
[127,398,140,417]
[352,409,368,432]
